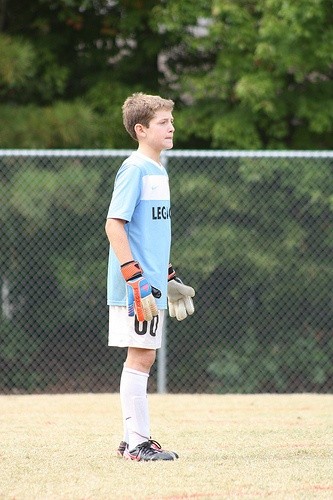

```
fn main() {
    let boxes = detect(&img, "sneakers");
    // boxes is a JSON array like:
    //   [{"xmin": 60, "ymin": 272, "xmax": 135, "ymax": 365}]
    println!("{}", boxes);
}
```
[{"xmin": 116, "ymin": 441, "xmax": 179, "ymax": 461}]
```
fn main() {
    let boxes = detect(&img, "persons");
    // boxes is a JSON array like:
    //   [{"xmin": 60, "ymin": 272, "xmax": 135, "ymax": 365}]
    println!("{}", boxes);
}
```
[{"xmin": 102, "ymin": 92, "xmax": 195, "ymax": 464}]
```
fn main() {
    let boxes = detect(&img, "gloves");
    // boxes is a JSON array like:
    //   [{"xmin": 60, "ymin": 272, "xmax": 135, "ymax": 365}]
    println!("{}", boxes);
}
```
[
  {"xmin": 120, "ymin": 261, "xmax": 162, "ymax": 322},
  {"xmin": 167, "ymin": 263, "xmax": 196, "ymax": 321}
]
